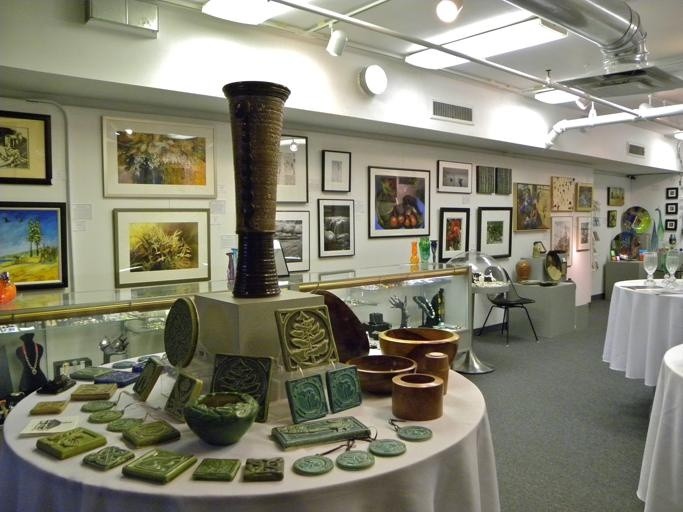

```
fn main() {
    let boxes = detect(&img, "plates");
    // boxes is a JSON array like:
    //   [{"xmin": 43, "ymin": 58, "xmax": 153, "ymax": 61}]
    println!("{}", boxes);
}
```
[
  {"xmin": 623, "ymin": 206, "xmax": 650, "ymax": 234},
  {"xmin": 544, "ymin": 249, "xmax": 562, "ymax": 282},
  {"xmin": 162, "ymin": 294, "xmax": 201, "ymax": 368},
  {"xmin": 309, "ymin": 287, "xmax": 370, "ymax": 363},
  {"xmin": 611, "ymin": 231, "xmax": 640, "ymax": 260}
]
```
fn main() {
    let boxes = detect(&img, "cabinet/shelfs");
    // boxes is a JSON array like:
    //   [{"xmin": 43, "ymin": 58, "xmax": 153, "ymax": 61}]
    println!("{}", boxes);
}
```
[{"xmin": 0, "ymin": 262, "xmax": 475, "ymax": 415}]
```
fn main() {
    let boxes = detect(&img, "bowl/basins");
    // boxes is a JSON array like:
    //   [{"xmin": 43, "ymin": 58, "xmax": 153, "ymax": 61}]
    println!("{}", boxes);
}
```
[{"xmin": 348, "ymin": 324, "xmax": 460, "ymax": 397}]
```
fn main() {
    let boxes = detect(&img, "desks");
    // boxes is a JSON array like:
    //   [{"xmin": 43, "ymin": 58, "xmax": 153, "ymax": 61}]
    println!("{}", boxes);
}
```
[{"xmin": 0, "ymin": 349, "xmax": 502, "ymax": 509}]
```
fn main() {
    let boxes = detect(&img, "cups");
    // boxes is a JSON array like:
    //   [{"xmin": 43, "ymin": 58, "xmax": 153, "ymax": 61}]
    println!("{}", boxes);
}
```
[
  {"xmin": 390, "ymin": 371, "xmax": 444, "ymax": 421},
  {"xmin": 184, "ymin": 391, "xmax": 258, "ymax": 447}
]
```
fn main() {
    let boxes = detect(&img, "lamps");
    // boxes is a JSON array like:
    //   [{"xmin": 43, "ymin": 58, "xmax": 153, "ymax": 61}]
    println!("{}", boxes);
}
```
[
  {"xmin": 434, "ymin": 0, "xmax": 462, "ymax": 24},
  {"xmin": 358, "ymin": 63, "xmax": 388, "ymax": 97},
  {"xmin": 403, "ymin": 16, "xmax": 570, "ymax": 71},
  {"xmin": 323, "ymin": 23, "xmax": 348, "ymax": 58}
]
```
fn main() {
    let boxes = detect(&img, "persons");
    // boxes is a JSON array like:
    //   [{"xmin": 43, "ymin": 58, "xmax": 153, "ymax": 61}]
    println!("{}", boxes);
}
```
[
  {"xmin": 388, "ymin": 294, "xmax": 410, "ymax": 329},
  {"xmin": 15, "ymin": 332, "xmax": 46, "ymax": 396}
]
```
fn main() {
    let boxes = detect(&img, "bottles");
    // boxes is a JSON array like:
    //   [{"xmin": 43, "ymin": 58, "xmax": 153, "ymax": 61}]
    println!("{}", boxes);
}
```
[
  {"xmin": 409, "ymin": 240, "xmax": 419, "ymax": 264},
  {"xmin": 532, "ymin": 241, "xmax": 541, "ymax": 258},
  {"xmin": 419, "ymin": 236, "xmax": 431, "ymax": 264}
]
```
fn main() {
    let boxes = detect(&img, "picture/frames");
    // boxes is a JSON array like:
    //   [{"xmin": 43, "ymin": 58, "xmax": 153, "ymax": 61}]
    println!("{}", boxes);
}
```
[
  {"xmin": 367, "ymin": 165, "xmax": 431, "ymax": 238},
  {"xmin": 436, "ymin": 160, "xmax": 591, "ymax": 267},
  {"xmin": 666, "ymin": 187, "xmax": 677, "ymax": 199},
  {"xmin": 100, "ymin": 115, "xmax": 217, "ymax": 201},
  {"xmin": 665, "ymin": 202, "xmax": 677, "ymax": 215},
  {"xmin": 665, "ymin": 219, "xmax": 677, "ymax": 230},
  {"xmin": 110, "ymin": 207, "xmax": 212, "ymax": 289},
  {"xmin": 0, "ymin": 110, "xmax": 52, "ymax": 186},
  {"xmin": 0, "ymin": 200, "xmax": 68, "ymax": 290},
  {"xmin": 275, "ymin": 134, "xmax": 356, "ymax": 271}
]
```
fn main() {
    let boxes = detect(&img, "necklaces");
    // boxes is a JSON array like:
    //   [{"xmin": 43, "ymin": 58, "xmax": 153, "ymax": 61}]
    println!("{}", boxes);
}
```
[
  {"xmin": 414, "ymin": 296, "xmax": 435, "ymax": 319},
  {"xmin": 21, "ymin": 342, "xmax": 40, "ymax": 376}
]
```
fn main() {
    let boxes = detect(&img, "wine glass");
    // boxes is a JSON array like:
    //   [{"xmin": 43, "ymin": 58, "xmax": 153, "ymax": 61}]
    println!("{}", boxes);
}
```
[
  {"xmin": 665, "ymin": 248, "xmax": 679, "ymax": 288},
  {"xmin": 431, "ymin": 239, "xmax": 438, "ymax": 264},
  {"xmin": 642, "ymin": 251, "xmax": 658, "ymax": 287}
]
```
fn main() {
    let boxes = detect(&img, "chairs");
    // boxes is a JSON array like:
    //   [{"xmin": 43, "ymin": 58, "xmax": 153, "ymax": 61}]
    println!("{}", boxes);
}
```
[{"xmin": 477, "ymin": 265, "xmax": 538, "ymax": 346}]
